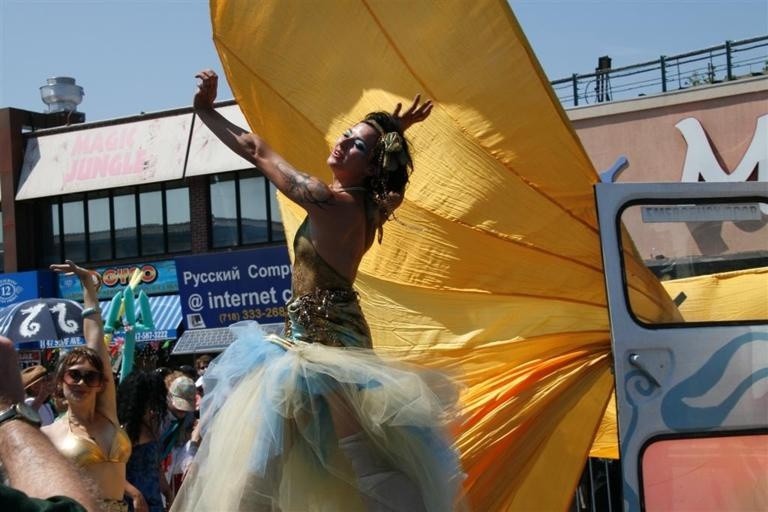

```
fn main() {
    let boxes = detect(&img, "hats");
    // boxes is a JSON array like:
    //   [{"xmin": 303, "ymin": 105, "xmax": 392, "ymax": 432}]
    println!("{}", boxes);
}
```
[
  {"xmin": 170, "ymin": 377, "xmax": 197, "ymax": 412},
  {"xmin": 20, "ymin": 363, "xmax": 49, "ymax": 389}
]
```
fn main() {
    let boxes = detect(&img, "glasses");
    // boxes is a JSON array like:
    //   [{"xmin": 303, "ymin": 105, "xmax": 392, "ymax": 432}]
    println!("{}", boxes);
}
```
[{"xmin": 62, "ymin": 368, "xmax": 105, "ymax": 387}]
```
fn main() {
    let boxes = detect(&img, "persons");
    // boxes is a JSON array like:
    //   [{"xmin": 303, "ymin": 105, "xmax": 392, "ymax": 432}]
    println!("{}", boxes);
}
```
[
  {"xmin": 1, "ymin": 260, "xmax": 213, "ymax": 511},
  {"xmin": 167, "ymin": 70, "xmax": 476, "ymax": 510}
]
